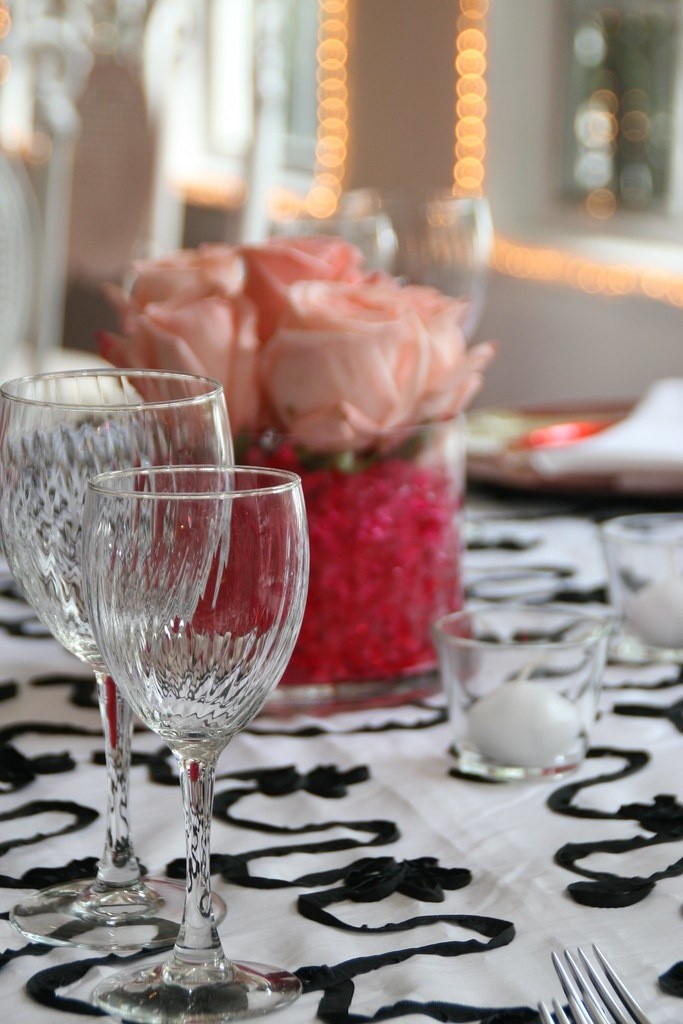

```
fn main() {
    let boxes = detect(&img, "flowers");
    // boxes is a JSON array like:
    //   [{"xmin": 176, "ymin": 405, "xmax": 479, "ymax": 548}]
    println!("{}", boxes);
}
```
[{"xmin": 97, "ymin": 231, "xmax": 498, "ymax": 469}]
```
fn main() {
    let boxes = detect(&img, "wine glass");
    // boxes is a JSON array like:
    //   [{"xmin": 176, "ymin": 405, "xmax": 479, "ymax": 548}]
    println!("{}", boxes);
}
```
[
  {"xmin": 0, "ymin": 369, "xmax": 236, "ymax": 953},
  {"xmin": 85, "ymin": 465, "xmax": 310, "ymax": 1024}
]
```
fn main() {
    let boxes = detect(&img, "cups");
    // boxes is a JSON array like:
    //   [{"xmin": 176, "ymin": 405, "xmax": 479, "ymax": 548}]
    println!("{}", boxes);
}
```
[
  {"xmin": 599, "ymin": 512, "xmax": 683, "ymax": 662},
  {"xmin": 430, "ymin": 604, "xmax": 613, "ymax": 783}
]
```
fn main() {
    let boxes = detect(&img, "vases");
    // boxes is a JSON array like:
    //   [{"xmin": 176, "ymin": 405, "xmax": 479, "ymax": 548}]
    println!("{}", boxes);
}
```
[{"xmin": 127, "ymin": 413, "xmax": 468, "ymax": 719}]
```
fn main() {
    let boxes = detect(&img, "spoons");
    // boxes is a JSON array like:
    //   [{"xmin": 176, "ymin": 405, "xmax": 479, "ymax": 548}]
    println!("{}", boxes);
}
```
[{"xmin": 537, "ymin": 943, "xmax": 652, "ymax": 1024}]
